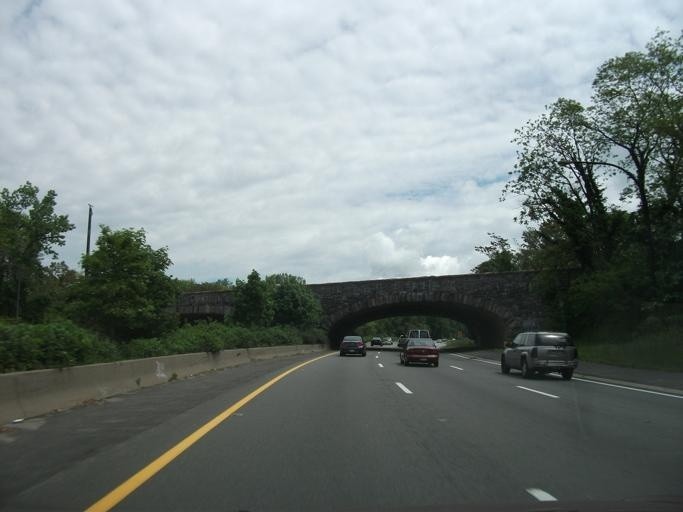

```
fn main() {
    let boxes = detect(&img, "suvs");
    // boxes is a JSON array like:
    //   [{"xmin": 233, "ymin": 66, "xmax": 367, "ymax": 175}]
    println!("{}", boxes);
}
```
[{"xmin": 499, "ymin": 329, "xmax": 580, "ymax": 382}]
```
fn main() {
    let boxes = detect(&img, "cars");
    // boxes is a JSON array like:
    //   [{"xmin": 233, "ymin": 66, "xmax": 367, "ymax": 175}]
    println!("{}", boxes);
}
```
[
  {"xmin": 369, "ymin": 328, "xmax": 447, "ymax": 368},
  {"xmin": 339, "ymin": 335, "xmax": 366, "ymax": 357}
]
